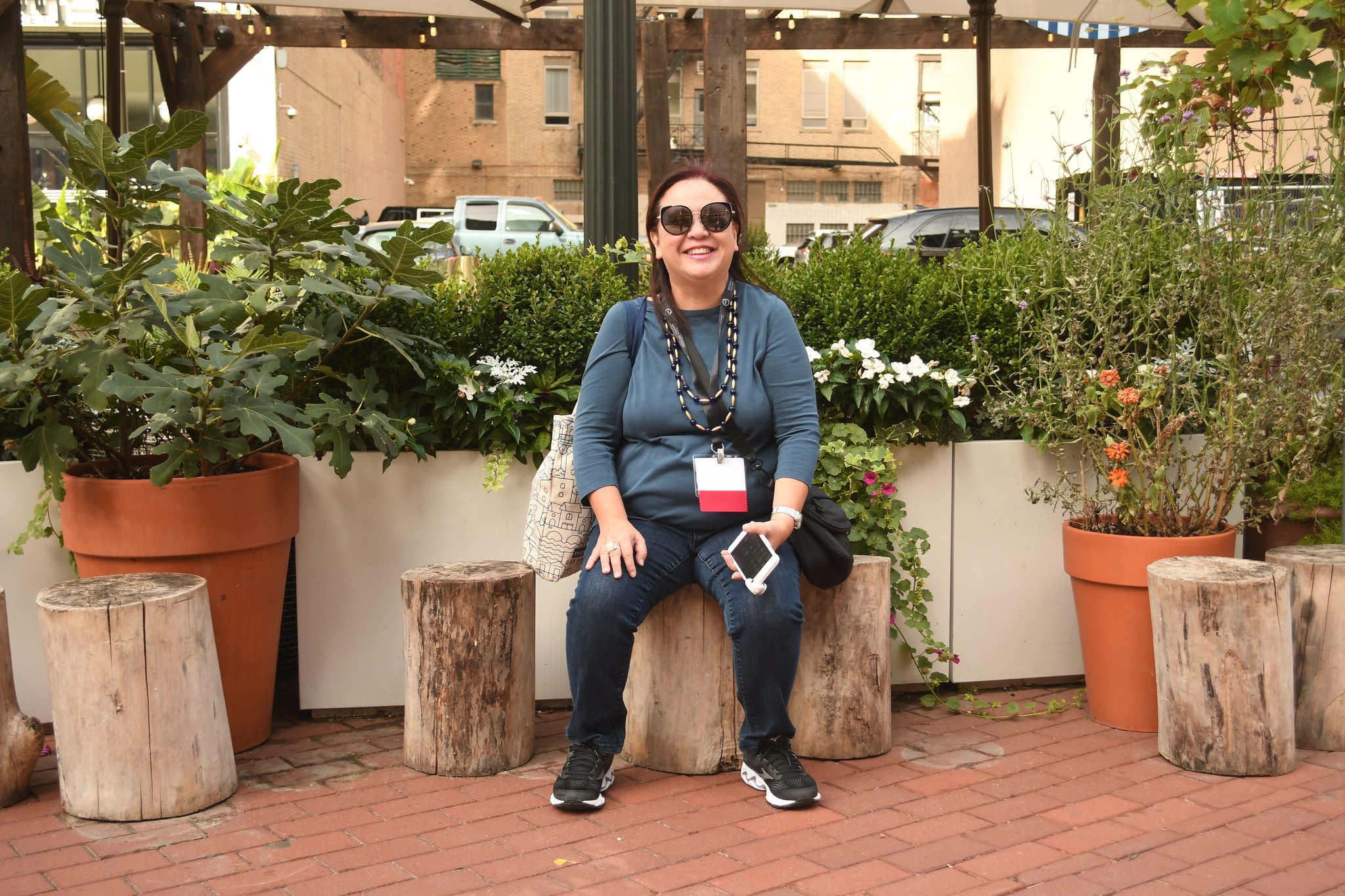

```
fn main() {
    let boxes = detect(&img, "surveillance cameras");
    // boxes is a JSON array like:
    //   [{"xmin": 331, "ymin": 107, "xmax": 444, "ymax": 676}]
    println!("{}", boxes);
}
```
[
  {"xmin": 408, "ymin": 180, "xmax": 416, "ymax": 185},
  {"xmin": 286, "ymin": 107, "xmax": 296, "ymax": 118}
]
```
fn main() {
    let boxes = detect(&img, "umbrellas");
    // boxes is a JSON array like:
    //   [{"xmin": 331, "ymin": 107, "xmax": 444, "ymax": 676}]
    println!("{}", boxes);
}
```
[
  {"xmin": 106, "ymin": 0, "xmax": 530, "ymax": 265},
  {"xmin": 520, "ymin": 0, "xmax": 1308, "ymax": 248}
]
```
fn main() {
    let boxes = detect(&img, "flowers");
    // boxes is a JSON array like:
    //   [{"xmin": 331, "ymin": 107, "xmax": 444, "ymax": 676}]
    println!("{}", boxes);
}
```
[{"xmin": 944, "ymin": 52, "xmax": 1345, "ymax": 549}]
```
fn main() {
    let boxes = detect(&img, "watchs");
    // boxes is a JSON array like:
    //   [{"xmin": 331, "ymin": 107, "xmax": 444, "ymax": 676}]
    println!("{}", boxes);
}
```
[{"xmin": 771, "ymin": 506, "xmax": 803, "ymax": 529}]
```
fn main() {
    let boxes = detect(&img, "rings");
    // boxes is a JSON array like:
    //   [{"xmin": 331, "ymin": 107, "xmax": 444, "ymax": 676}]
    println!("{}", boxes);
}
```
[{"xmin": 606, "ymin": 541, "xmax": 621, "ymax": 553}]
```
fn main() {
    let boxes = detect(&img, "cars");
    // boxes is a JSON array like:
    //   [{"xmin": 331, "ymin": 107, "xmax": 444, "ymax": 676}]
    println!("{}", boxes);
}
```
[{"xmin": 353, "ymin": 218, "xmax": 455, "ymax": 267}]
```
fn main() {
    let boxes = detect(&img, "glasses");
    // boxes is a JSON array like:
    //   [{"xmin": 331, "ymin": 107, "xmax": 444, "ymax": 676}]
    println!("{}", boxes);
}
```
[{"xmin": 655, "ymin": 201, "xmax": 736, "ymax": 236}]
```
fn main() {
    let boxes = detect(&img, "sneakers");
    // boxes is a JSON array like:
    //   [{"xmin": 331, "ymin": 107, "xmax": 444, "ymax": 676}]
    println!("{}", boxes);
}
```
[
  {"xmin": 550, "ymin": 743, "xmax": 615, "ymax": 810},
  {"xmin": 741, "ymin": 741, "xmax": 821, "ymax": 809}
]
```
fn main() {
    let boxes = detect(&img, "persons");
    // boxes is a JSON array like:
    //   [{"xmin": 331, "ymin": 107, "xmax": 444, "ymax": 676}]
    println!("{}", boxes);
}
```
[{"xmin": 549, "ymin": 163, "xmax": 821, "ymax": 809}]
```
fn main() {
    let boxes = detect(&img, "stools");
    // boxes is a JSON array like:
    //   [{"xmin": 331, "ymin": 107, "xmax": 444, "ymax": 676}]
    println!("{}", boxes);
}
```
[
  {"xmin": 400, "ymin": 557, "xmax": 539, "ymax": 781},
  {"xmin": 1148, "ymin": 555, "xmax": 1296, "ymax": 775},
  {"xmin": 1263, "ymin": 542, "xmax": 1345, "ymax": 754},
  {"xmin": 618, "ymin": 577, "xmax": 745, "ymax": 776},
  {"xmin": 35, "ymin": 562, "xmax": 246, "ymax": 820},
  {"xmin": 784, "ymin": 549, "xmax": 894, "ymax": 762}
]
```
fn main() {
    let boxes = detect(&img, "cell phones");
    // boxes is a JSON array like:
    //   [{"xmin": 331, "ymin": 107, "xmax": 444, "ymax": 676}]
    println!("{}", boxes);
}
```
[{"xmin": 728, "ymin": 522, "xmax": 780, "ymax": 587}]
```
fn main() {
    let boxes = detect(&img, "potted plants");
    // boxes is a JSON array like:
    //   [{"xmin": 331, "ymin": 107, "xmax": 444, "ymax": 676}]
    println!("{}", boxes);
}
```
[{"xmin": 0, "ymin": 55, "xmax": 498, "ymax": 753}]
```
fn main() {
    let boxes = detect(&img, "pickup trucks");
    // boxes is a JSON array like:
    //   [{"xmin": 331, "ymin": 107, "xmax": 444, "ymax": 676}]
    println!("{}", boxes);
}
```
[{"xmin": 453, "ymin": 196, "xmax": 585, "ymax": 258}]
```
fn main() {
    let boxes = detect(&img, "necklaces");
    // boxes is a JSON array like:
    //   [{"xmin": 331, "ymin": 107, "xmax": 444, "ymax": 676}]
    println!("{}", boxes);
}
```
[{"xmin": 663, "ymin": 288, "xmax": 740, "ymax": 433}]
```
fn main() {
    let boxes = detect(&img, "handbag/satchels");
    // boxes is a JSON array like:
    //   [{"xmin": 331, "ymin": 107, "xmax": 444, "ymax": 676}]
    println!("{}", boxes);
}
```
[
  {"xmin": 762, "ymin": 475, "xmax": 855, "ymax": 590},
  {"xmin": 523, "ymin": 391, "xmax": 596, "ymax": 582}
]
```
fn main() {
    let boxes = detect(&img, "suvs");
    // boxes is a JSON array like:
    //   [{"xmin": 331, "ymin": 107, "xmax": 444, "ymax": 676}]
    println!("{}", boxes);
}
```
[{"xmin": 791, "ymin": 204, "xmax": 1091, "ymax": 266}]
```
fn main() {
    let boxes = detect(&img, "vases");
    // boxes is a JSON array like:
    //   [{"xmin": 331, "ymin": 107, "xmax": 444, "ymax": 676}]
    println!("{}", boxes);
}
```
[
  {"xmin": 1060, "ymin": 511, "xmax": 1238, "ymax": 736},
  {"xmin": 1237, "ymin": 487, "xmax": 1345, "ymax": 561}
]
